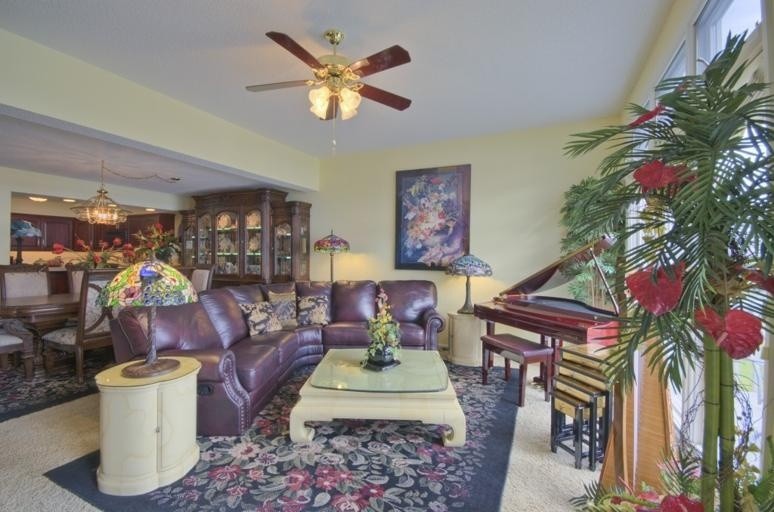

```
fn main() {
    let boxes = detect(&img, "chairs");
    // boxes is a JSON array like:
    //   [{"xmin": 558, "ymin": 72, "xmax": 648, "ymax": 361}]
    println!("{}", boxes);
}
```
[
  {"xmin": 31, "ymin": 264, "xmax": 121, "ymax": 385},
  {"xmin": 0, "ymin": 317, "xmax": 38, "ymax": 386},
  {"xmin": 0, "ymin": 261, "xmax": 67, "ymax": 338},
  {"xmin": 61, "ymin": 262, "xmax": 87, "ymax": 299},
  {"xmin": 184, "ymin": 260, "xmax": 217, "ymax": 295}
]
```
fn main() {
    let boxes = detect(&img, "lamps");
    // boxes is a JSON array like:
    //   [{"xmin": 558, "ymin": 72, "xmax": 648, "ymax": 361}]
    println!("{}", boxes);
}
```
[
  {"xmin": 443, "ymin": 243, "xmax": 495, "ymax": 315},
  {"xmin": 91, "ymin": 259, "xmax": 201, "ymax": 378},
  {"xmin": 306, "ymin": 73, "xmax": 360, "ymax": 119},
  {"xmin": 68, "ymin": 157, "xmax": 133, "ymax": 227},
  {"xmin": 9, "ymin": 219, "xmax": 43, "ymax": 264},
  {"xmin": 315, "ymin": 230, "xmax": 350, "ymax": 284}
]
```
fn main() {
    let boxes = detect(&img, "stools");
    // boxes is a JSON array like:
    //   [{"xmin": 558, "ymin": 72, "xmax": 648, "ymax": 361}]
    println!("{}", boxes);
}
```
[{"xmin": 479, "ymin": 330, "xmax": 552, "ymax": 407}]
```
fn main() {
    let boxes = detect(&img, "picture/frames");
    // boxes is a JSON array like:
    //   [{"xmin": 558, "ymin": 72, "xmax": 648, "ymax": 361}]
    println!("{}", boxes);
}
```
[{"xmin": 391, "ymin": 163, "xmax": 472, "ymax": 272}]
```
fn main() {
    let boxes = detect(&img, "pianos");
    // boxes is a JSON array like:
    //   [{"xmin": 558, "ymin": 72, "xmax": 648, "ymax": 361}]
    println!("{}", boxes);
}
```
[{"xmin": 474, "ymin": 236, "xmax": 623, "ymax": 386}]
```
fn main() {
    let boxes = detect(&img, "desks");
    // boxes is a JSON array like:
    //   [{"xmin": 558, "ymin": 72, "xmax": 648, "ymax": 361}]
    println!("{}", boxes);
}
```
[
  {"xmin": 1, "ymin": 291, "xmax": 85, "ymax": 381},
  {"xmin": 550, "ymin": 343, "xmax": 625, "ymax": 469}
]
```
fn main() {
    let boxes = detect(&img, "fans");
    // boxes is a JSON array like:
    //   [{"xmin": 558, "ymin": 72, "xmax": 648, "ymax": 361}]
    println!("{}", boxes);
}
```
[{"xmin": 244, "ymin": 28, "xmax": 416, "ymax": 119}]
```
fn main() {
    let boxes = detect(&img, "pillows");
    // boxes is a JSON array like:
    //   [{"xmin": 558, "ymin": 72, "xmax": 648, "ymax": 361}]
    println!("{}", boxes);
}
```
[
  {"xmin": 266, "ymin": 290, "xmax": 297, "ymax": 329},
  {"xmin": 296, "ymin": 292, "xmax": 328, "ymax": 327},
  {"xmin": 236, "ymin": 300, "xmax": 281, "ymax": 338}
]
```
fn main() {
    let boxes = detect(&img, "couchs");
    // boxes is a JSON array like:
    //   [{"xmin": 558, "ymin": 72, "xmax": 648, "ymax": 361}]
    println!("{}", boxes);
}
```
[{"xmin": 108, "ymin": 278, "xmax": 444, "ymax": 438}]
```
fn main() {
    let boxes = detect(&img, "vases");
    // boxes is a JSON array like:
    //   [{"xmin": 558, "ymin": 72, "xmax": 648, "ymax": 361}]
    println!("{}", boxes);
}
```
[{"xmin": 364, "ymin": 346, "xmax": 396, "ymax": 364}]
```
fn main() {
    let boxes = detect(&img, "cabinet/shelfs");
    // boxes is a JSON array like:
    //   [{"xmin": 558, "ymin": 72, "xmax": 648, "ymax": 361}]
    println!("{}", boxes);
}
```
[
  {"xmin": 444, "ymin": 312, "xmax": 488, "ymax": 369},
  {"xmin": 190, "ymin": 187, "xmax": 288, "ymax": 289},
  {"xmin": 73, "ymin": 212, "xmax": 98, "ymax": 255},
  {"xmin": 124, "ymin": 211, "xmax": 175, "ymax": 251},
  {"xmin": 271, "ymin": 199, "xmax": 311, "ymax": 284},
  {"xmin": 92, "ymin": 353, "xmax": 202, "ymax": 497},
  {"xmin": 93, "ymin": 210, "xmax": 126, "ymax": 251},
  {"xmin": 178, "ymin": 208, "xmax": 195, "ymax": 275},
  {"xmin": 10, "ymin": 210, "xmax": 72, "ymax": 251}
]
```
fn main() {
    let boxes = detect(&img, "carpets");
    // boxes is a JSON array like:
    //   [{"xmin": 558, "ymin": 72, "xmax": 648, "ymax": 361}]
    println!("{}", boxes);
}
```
[
  {"xmin": 40, "ymin": 357, "xmax": 532, "ymax": 512},
  {"xmin": 1, "ymin": 337, "xmax": 114, "ymax": 421}
]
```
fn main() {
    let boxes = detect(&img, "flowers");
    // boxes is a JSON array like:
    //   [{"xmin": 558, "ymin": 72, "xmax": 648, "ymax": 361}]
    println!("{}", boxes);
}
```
[
  {"xmin": 362, "ymin": 288, "xmax": 402, "ymax": 350},
  {"xmin": 50, "ymin": 237, "xmax": 134, "ymax": 271},
  {"xmin": 613, "ymin": 102, "xmax": 771, "ymax": 510},
  {"xmin": 134, "ymin": 221, "xmax": 183, "ymax": 260}
]
